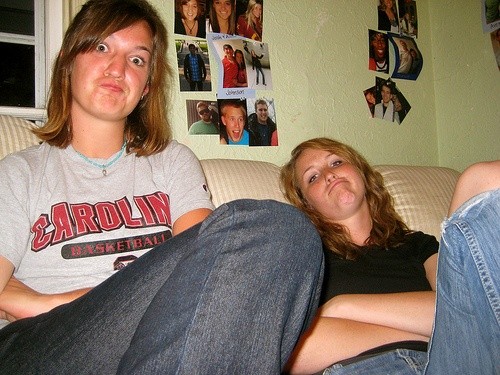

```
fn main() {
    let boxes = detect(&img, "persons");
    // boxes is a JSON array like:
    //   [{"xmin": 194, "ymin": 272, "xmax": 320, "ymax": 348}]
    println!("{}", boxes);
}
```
[
  {"xmin": 369, "ymin": 33, "xmax": 389, "ymax": 74},
  {"xmin": 175, "ymin": 0, "xmax": 206, "ymax": 38},
  {"xmin": 365, "ymin": 83, "xmax": 407, "ymax": 126},
  {"xmin": 189, "ymin": 102, "xmax": 220, "ymax": 134},
  {"xmin": 251, "ymin": 50, "xmax": 267, "ymax": 86},
  {"xmin": 206, "ymin": 0, "xmax": 236, "ymax": 36},
  {"xmin": 279, "ymin": 137, "xmax": 500, "ymax": 375},
  {"xmin": 0, "ymin": 0, "xmax": 324, "ymax": 375},
  {"xmin": 396, "ymin": 40, "xmax": 421, "ymax": 76},
  {"xmin": 222, "ymin": 44, "xmax": 248, "ymax": 88},
  {"xmin": 377, "ymin": 0, "xmax": 415, "ymax": 36},
  {"xmin": 219, "ymin": 102, "xmax": 249, "ymax": 145},
  {"xmin": 184, "ymin": 44, "xmax": 207, "ymax": 91},
  {"xmin": 247, "ymin": 99, "xmax": 278, "ymax": 146},
  {"xmin": 236, "ymin": 0, "xmax": 262, "ymax": 42}
]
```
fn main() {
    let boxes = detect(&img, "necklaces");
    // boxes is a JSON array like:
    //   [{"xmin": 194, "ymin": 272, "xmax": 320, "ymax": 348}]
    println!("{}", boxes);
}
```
[
  {"xmin": 184, "ymin": 20, "xmax": 196, "ymax": 34},
  {"xmin": 75, "ymin": 138, "xmax": 127, "ymax": 175}
]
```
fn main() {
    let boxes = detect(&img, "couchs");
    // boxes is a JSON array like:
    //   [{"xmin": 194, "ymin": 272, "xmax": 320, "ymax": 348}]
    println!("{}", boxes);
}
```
[{"xmin": 0, "ymin": 114, "xmax": 461, "ymax": 242}]
